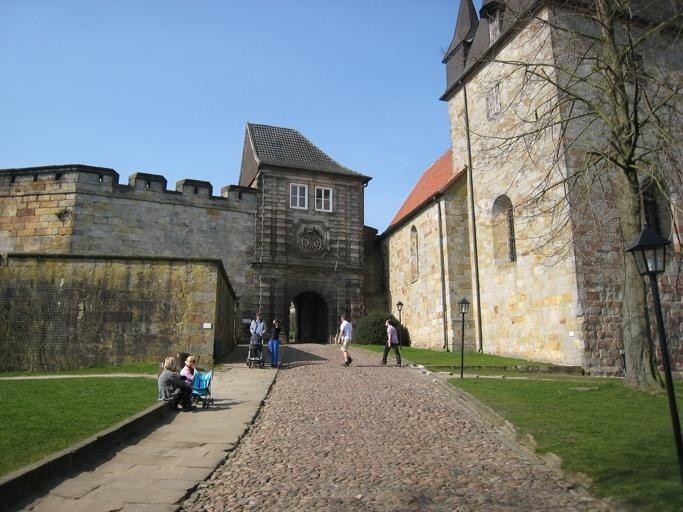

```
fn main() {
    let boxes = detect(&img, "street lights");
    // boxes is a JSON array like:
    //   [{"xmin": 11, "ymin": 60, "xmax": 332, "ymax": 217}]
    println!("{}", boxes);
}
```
[
  {"xmin": 395, "ymin": 300, "xmax": 404, "ymax": 350},
  {"xmin": 456, "ymin": 297, "xmax": 471, "ymax": 379},
  {"xmin": 624, "ymin": 225, "xmax": 682, "ymax": 483}
]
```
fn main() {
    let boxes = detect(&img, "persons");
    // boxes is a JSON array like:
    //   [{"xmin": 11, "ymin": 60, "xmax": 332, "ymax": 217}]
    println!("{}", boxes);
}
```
[
  {"xmin": 249, "ymin": 312, "xmax": 266, "ymax": 359},
  {"xmin": 378, "ymin": 319, "xmax": 402, "ymax": 367},
  {"xmin": 336, "ymin": 313, "xmax": 353, "ymax": 367},
  {"xmin": 157, "ymin": 356, "xmax": 196, "ymax": 412},
  {"xmin": 178, "ymin": 356, "xmax": 199, "ymax": 402},
  {"xmin": 267, "ymin": 318, "xmax": 282, "ymax": 368}
]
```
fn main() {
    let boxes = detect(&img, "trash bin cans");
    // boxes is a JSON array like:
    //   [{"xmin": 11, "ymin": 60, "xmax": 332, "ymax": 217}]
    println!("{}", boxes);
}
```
[{"xmin": 175, "ymin": 353, "xmax": 189, "ymax": 369}]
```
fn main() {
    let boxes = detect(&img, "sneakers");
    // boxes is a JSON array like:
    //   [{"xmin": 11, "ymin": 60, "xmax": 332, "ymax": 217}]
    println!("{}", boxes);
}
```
[
  {"xmin": 395, "ymin": 364, "xmax": 401, "ymax": 367},
  {"xmin": 380, "ymin": 361, "xmax": 387, "ymax": 364}
]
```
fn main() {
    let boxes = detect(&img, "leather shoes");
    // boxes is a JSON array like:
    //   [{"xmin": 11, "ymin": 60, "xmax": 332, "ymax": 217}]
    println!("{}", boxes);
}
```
[
  {"xmin": 177, "ymin": 404, "xmax": 196, "ymax": 412},
  {"xmin": 341, "ymin": 357, "xmax": 352, "ymax": 366}
]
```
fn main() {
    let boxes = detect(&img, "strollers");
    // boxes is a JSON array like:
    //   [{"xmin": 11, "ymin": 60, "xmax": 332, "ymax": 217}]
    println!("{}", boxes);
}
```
[
  {"xmin": 190, "ymin": 369, "xmax": 215, "ymax": 409},
  {"xmin": 247, "ymin": 332, "xmax": 265, "ymax": 368}
]
